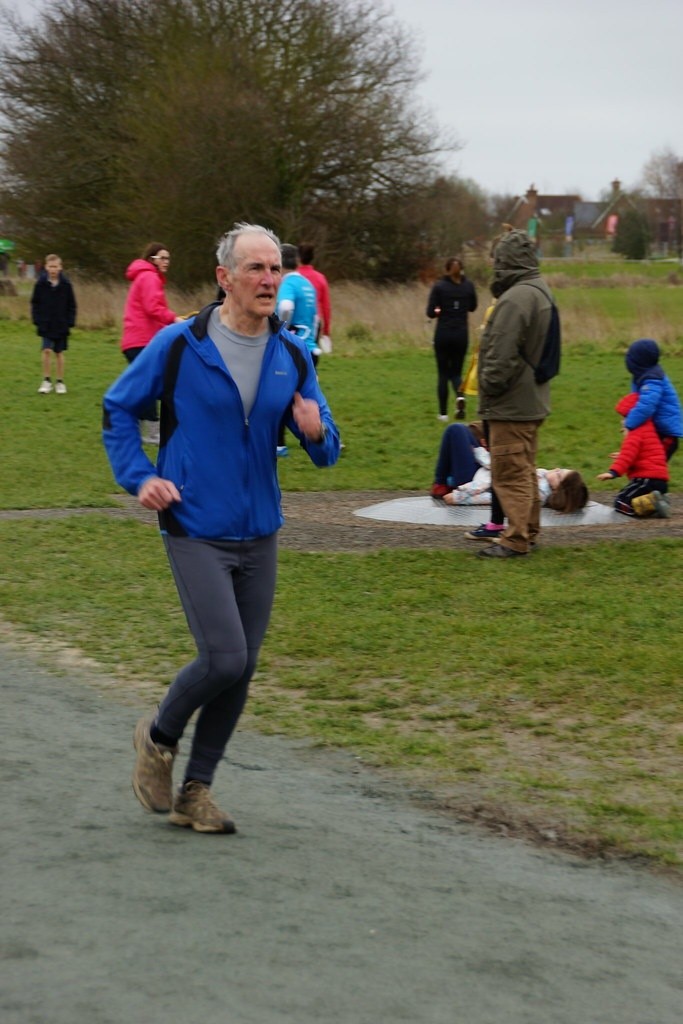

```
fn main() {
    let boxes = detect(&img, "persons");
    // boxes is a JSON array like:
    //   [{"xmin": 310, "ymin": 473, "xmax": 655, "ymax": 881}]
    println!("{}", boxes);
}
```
[
  {"xmin": 121, "ymin": 242, "xmax": 200, "ymax": 446},
  {"xmin": 102, "ymin": 221, "xmax": 341, "ymax": 832},
  {"xmin": 426, "ymin": 258, "xmax": 478, "ymax": 421},
  {"xmin": 477, "ymin": 231, "xmax": 557, "ymax": 560},
  {"xmin": 596, "ymin": 392, "xmax": 671, "ymax": 520},
  {"xmin": 464, "ymin": 238, "xmax": 506, "ymax": 542},
  {"xmin": 297, "ymin": 241, "xmax": 332, "ymax": 367},
  {"xmin": 430, "ymin": 425, "xmax": 589, "ymax": 516},
  {"xmin": 274, "ymin": 245, "xmax": 346, "ymax": 457},
  {"xmin": 621, "ymin": 338, "xmax": 683, "ymax": 462},
  {"xmin": 30, "ymin": 254, "xmax": 77, "ymax": 394}
]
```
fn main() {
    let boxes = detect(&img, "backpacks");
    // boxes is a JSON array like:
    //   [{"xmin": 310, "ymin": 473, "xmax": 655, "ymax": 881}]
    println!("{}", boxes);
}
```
[{"xmin": 515, "ymin": 280, "xmax": 562, "ymax": 386}]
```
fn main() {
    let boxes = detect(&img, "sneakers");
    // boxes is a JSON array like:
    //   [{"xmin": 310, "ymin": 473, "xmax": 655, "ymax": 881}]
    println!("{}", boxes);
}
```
[
  {"xmin": 132, "ymin": 716, "xmax": 176, "ymax": 816},
  {"xmin": 170, "ymin": 781, "xmax": 234, "ymax": 834},
  {"xmin": 463, "ymin": 524, "xmax": 505, "ymax": 542},
  {"xmin": 477, "ymin": 539, "xmax": 538, "ymax": 558}
]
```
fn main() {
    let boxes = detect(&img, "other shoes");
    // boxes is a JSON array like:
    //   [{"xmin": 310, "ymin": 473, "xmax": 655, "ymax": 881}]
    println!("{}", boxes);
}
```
[
  {"xmin": 436, "ymin": 414, "xmax": 448, "ymax": 422},
  {"xmin": 56, "ymin": 382, "xmax": 66, "ymax": 393},
  {"xmin": 340, "ymin": 439, "xmax": 345, "ymax": 450},
  {"xmin": 38, "ymin": 379, "xmax": 53, "ymax": 392},
  {"xmin": 651, "ymin": 490, "xmax": 673, "ymax": 518},
  {"xmin": 139, "ymin": 418, "xmax": 160, "ymax": 444},
  {"xmin": 454, "ymin": 397, "xmax": 466, "ymax": 421},
  {"xmin": 432, "ymin": 485, "xmax": 451, "ymax": 499},
  {"xmin": 277, "ymin": 447, "xmax": 289, "ymax": 457}
]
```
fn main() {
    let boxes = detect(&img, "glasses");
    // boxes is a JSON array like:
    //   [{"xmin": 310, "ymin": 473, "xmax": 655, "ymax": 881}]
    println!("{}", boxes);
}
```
[{"xmin": 150, "ymin": 255, "xmax": 171, "ymax": 261}]
